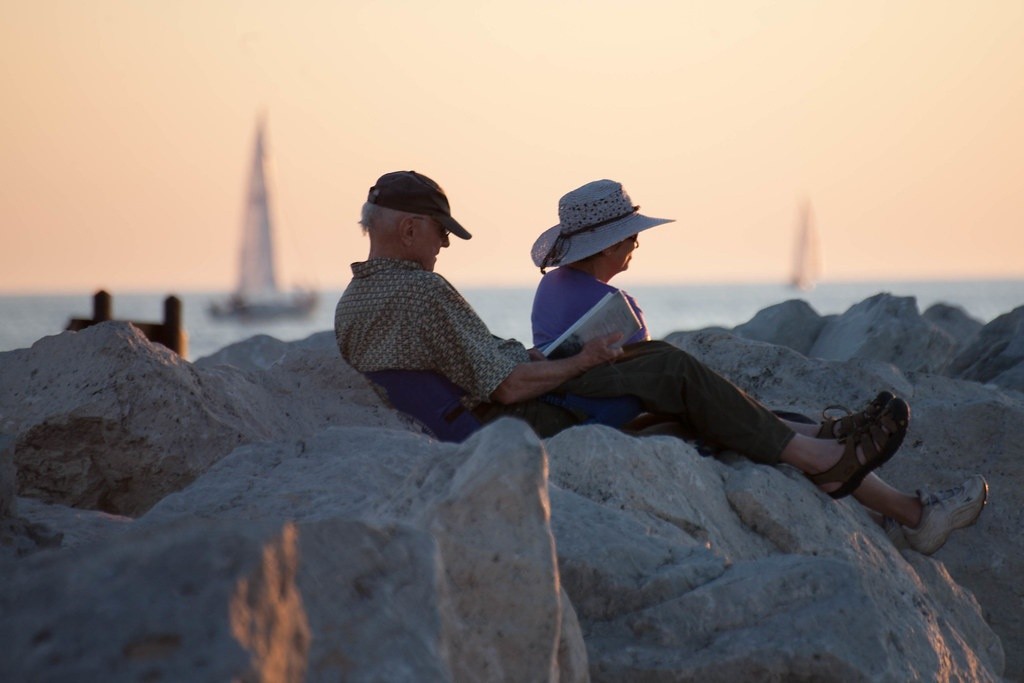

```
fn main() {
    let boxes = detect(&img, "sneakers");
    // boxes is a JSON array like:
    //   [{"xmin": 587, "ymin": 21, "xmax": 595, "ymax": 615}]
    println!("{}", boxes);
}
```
[
  {"xmin": 885, "ymin": 518, "xmax": 908, "ymax": 549},
  {"xmin": 900, "ymin": 475, "xmax": 988, "ymax": 555}
]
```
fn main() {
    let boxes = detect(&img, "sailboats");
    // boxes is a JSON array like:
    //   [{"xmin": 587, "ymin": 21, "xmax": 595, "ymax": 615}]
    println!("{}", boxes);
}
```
[{"xmin": 200, "ymin": 112, "xmax": 323, "ymax": 327}]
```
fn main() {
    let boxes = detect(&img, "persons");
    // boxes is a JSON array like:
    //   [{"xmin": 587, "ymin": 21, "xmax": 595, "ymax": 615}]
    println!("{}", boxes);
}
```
[
  {"xmin": 531, "ymin": 180, "xmax": 989, "ymax": 555},
  {"xmin": 333, "ymin": 170, "xmax": 912, "ymax": 501}
]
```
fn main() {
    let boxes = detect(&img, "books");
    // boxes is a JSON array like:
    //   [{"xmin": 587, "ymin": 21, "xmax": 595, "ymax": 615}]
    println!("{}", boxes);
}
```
[{"xmin": 538, "ymin": 290, "xmax": 644, "ymax": 361}]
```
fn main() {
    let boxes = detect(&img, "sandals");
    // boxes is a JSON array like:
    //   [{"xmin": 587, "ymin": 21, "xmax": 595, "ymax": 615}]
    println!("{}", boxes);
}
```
[
  {"xmin": 804, "ymin": 397, "xmax": 911, "ymax": 499},
  {"xmin": 816, "ymin": 390, "xmax": 895, "ymax": 440}
]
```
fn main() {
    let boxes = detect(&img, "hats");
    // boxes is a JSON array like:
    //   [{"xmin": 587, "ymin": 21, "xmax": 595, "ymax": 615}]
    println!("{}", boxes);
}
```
[
  {"xmin": 369, "ymin": 170, "xmax": 473, "ymax": 239},
  {"xmin": 531, "ymin": 179, "xmax": 675, "ymax": 274}
]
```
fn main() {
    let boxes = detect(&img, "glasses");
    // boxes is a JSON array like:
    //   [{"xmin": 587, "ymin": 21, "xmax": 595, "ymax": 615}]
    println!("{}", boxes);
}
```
[{"xmin": 413, "ymin": 216, "xmax": 450, "ymax": 237}]
